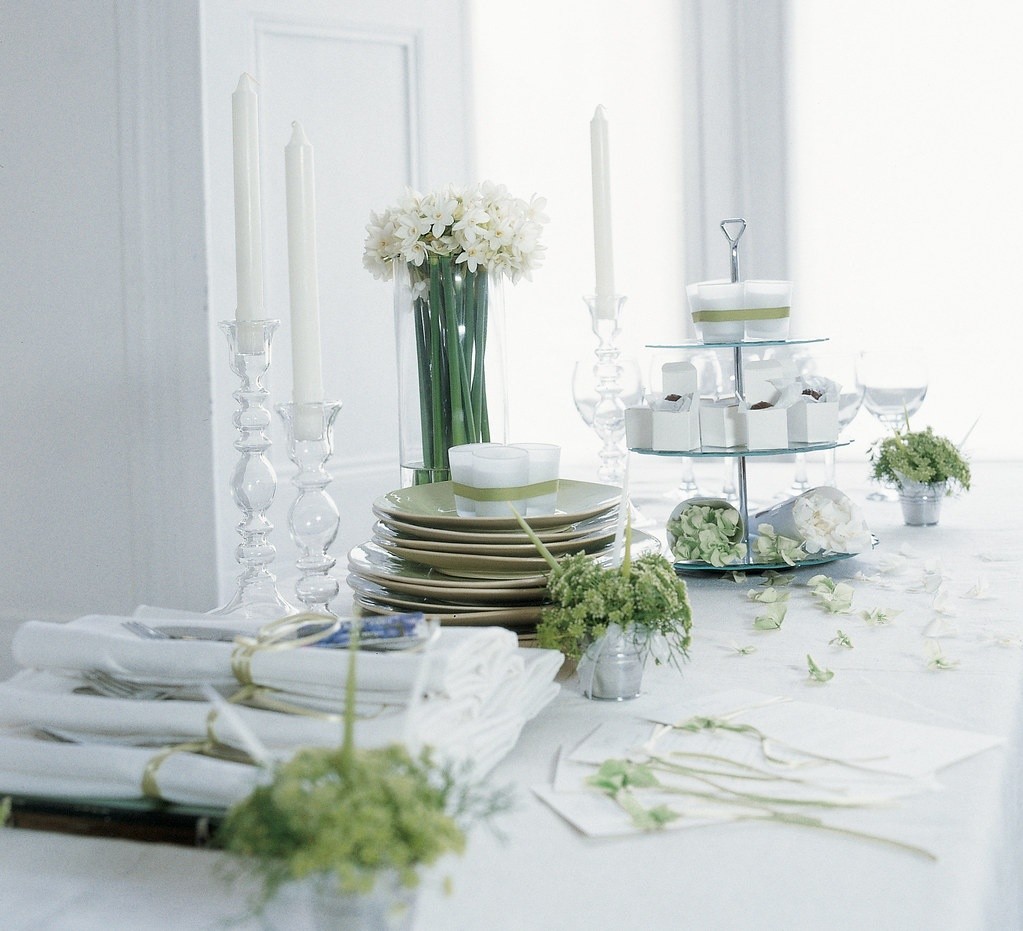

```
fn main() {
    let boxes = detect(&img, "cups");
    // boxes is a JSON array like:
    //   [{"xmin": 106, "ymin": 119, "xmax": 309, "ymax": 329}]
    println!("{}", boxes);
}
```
[
  {"xmin": 747, "ymin": 486, "xmax": 869, "ymax": 554},
  {"xmin": 685, "ymin": 278, "xmax": 793, "ymax": 346},
  {"xmin": 447, "ymin": 439, "xmax": 561, "ymax": 519},
  {"xmin": 665, "ymin": 496, "xmax": 745, "ymax": 561}
]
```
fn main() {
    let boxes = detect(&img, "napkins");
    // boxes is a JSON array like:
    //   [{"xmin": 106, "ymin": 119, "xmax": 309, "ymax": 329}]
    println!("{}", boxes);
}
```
[{"xmin": 0, "ymin": 603, "xmax": 566, "ymax": 809}]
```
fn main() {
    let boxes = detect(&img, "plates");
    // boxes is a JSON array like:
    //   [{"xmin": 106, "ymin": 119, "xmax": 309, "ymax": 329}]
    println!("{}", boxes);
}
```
[
  {"xmin": 346, "ymin": 480, "xmax": 663, "ymax": 640},
  {"xmin": 672, "ymin": 532, "xmax": 880, "ymax": 573}
]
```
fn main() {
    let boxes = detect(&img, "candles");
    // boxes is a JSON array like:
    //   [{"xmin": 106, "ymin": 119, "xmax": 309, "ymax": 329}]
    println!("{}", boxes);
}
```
[
  {"xmin": 232, "ymin": 74, "xmax": 263, "ymax": 353},
  {"xmin": 283, "ymin": 120, "xmax": 326, "ymax": 436},
  {"xmin": 589, "ymin": 105, "xmax": 616, "ymax": 318}
]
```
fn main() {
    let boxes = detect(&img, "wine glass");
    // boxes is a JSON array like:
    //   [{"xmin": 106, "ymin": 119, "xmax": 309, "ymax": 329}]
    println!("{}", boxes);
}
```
[
  {"xmin": 852, "ymin": 342, "xmax": 930, "ymax": 501},
  {"xmin": 571, "ymin": 346, "xmax": 645, "ymax": 521},
  {"xmin": 646, "ymin": 340, "xmax": 867, "ymax": 512}
]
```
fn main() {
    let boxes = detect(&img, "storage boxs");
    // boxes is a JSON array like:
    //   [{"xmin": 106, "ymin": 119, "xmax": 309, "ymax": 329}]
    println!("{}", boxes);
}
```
[{"xmin": 625, "ymin": 360, "xmax": 842, "ymax": 452}]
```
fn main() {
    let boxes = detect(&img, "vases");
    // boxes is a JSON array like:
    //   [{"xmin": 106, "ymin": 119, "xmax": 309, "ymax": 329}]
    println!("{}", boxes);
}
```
[
  {"xmin": 583, "ymin": 620, "xmax": 653, "ymax": 702},
  {"xmin": 891, "ymin": 469, "xmax": 947, "ymax": 526},
  {"xmin": 394, "ymin": 256, "xmax": 506, "ymax": 490},
  {"xmin": 303, "ymin": 869, "xmax": 420, "ymax": 931}
]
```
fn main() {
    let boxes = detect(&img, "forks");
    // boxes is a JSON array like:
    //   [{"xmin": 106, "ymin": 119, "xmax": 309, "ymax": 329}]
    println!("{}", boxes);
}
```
[
  {"xmin": 121, "ymin": 617, "xmax": 235, "ymax": 645},
  {"xmin": 82, "ymin": 666, "xmax": 339, "ymax": 720}
]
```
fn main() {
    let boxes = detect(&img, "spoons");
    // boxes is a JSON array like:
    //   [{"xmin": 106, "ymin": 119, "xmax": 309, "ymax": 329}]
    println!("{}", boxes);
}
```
[{"xmin": 36, "ymin": 723, "xmax": 233, "ymax": 749}]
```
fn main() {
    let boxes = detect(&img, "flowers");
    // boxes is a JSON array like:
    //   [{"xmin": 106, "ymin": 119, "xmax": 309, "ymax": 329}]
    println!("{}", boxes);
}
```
[
  {"xmin": 199, "ymin": 600, "xmax": 470, "ymax": 912},
  {"xmin": 507, "ymin": 490, "xmax": 693, "ymax": 677},
  {"xmin": 360, "ymin": 180, "xmax": 550, "ymax": 485},
  {"xmin": 865, "ymin": 397, "xmax": 979, "ymax": 495},
  {"xmin": 588, "ymin": 715, "xmax": 938, "ymax": 864},
  {"xmin": 669, "ymin": 494, "xmax": 1023, "ymax": 684}
]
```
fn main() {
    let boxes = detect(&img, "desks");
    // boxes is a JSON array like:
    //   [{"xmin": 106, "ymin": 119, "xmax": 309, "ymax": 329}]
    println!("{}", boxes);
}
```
[{"xmin": 0, "ymin": 476, "xmax": 1022, "ymax": 929}]
